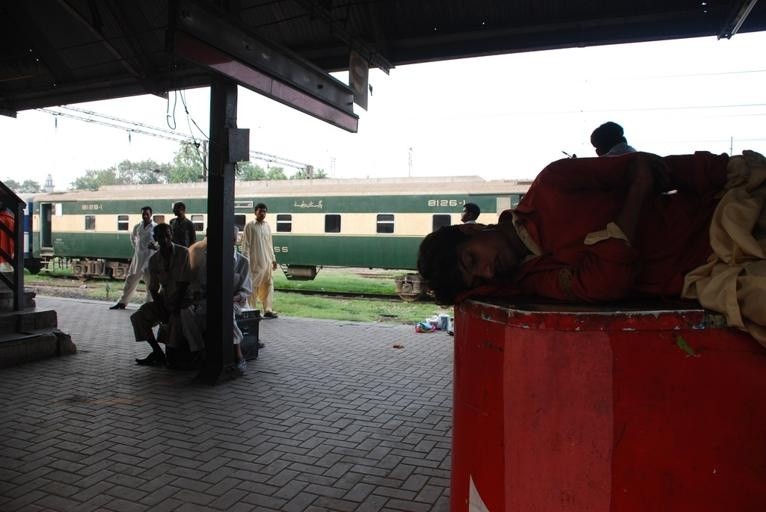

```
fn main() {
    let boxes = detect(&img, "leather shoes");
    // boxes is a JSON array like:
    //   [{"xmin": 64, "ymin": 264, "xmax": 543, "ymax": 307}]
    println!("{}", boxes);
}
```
[
  {"xmin": 110, "ymin": 303, "xmax": 125, "ymax": 309},
  {"xmin": 135, "ymin": 352, "xmax": 165, "ymax": 362}
]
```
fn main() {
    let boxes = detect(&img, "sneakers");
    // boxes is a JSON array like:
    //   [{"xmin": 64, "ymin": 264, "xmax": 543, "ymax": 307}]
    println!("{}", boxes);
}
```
[
  {"xmin": 238, "ymin": 359, "xmax": 247, "ymax": 369},
  {"xmin": 264, "ymin": 312, "xmax": 277, "ymax": 318}
]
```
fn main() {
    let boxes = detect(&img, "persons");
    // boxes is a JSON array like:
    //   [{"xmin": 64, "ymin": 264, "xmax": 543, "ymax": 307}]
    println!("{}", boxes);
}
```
[
  {"xmin": 168, "ymin": 202, "xmax": 197, "ymax": 249},
  {"xmin": 417, "ymin": 152, "xmax": 766, "ymax": 351},
  {"xmin": 182, "ymin": 226, "xmax": 249, "ymax": 382},
  {"xmin": 165, "ymin": 226, "xmax": 208, "ymax": 372},
  {"xmin": 242, "ymin": 204, "xmax": 279, "ymax": 320},
  {"xmin": 130, "ymin": 222, "xmax": 191, "ymax": 369},
  {"xmin": 109, "ymin": 207, "xmax": 161, "ymax": 310},
  {"xmin": 567, "ymin": 121, "xmax": 639, "ymax": 160},
  {"xmin": 0, "ymin": 197, "xmax": 16, "ymax": 273},
  {"xmin": 459, "ymin": 202, "xmax": 483, "ymax": 226}
]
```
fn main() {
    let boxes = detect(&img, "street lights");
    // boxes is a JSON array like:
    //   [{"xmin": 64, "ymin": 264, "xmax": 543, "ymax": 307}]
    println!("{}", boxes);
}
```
[{"xmin": 408, "ymin": 147, "xmax": 413, "ymax": 176}]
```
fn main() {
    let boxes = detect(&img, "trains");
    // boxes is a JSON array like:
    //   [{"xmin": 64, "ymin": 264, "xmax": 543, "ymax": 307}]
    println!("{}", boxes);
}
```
[{"xmin": 12, "ymin": 168, "xmax": 534, "ymax": 306}]
